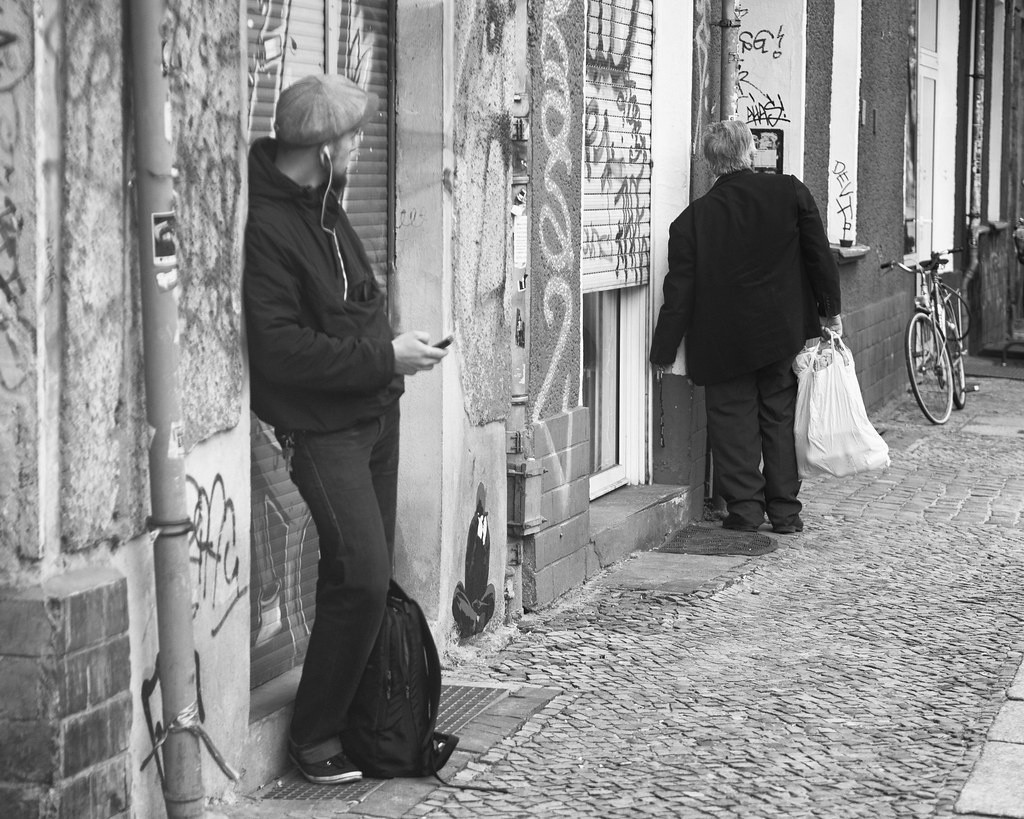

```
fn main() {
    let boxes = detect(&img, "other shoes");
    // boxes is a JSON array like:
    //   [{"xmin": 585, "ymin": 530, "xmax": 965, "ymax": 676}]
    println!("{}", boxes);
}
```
[
  {"xmin": 773, "ymin": 514, "xmax": 804, "ymax": 533},
  {"xmin": 723, "ymin": 514, "xmax": 759, "ymax": 531}
]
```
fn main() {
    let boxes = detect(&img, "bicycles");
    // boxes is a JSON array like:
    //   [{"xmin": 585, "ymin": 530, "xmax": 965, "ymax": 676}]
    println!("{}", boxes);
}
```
[{"xmin": 879, "ymin": 247, "xmax": 979, "ymax": 426}]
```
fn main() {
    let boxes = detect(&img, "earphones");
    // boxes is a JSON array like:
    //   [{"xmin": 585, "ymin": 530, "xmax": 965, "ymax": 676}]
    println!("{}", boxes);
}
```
[{"xmin": 323, "ymin": 145, "xmax": 330, "ymax": 159}]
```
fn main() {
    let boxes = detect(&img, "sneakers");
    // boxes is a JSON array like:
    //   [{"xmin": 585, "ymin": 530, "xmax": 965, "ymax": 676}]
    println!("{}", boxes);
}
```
[{"xmin": 288, "ymin": 734, "xmax": 361, "ymax": 785}]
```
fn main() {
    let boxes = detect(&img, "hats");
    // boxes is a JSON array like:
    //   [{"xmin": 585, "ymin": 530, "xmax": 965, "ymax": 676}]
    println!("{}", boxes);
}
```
[{"xmin": 274, "ymin": 74, "xmax": 379, "ymax": 145}]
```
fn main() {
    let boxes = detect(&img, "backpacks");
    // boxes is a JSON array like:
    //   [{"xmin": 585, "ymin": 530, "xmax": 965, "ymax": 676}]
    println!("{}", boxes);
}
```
[{"xmin": 339, "ymin": 578, "xmax": 459, "ymax": 779}]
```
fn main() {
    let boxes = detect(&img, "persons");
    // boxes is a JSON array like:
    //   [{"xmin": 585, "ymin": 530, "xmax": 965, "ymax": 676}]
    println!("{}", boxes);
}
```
[
  {"xmin": 243, "ymin": 74, "xmax": 447, "ymax": 783},
  {"xmin": 649, "ymin": 121, "xmax": 842, "ymax": 533}
]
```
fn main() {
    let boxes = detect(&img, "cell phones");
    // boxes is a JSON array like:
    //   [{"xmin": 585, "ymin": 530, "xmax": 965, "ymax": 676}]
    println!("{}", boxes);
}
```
[{"xmin": 433, "ymin": 335, "xmax": 453, "ymax": 349}]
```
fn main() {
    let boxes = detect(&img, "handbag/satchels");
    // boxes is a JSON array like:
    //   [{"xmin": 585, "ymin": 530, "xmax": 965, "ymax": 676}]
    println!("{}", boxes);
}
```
[{"xmin": 794, "ymin": 330, "xmax": 890, "ymax": 483}]
